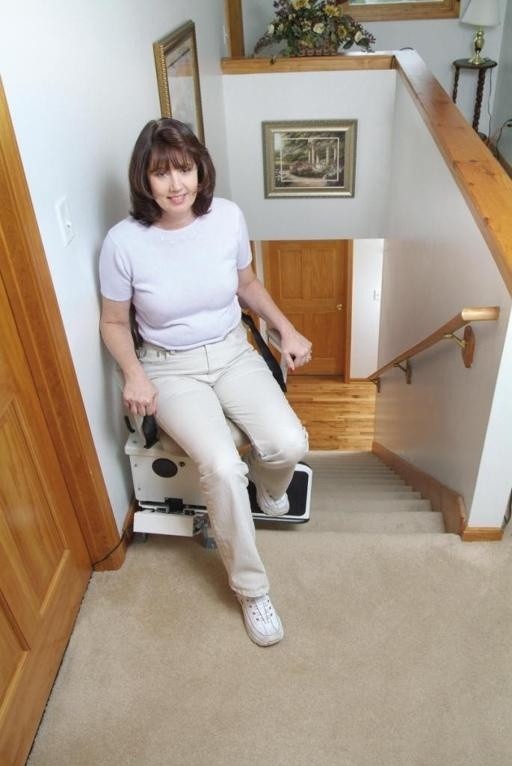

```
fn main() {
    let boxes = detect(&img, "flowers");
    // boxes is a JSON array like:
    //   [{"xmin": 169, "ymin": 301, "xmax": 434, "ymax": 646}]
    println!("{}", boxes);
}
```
[{"xmin": 247, "ymin": 0, "xmax": 376, "ymax": 66}]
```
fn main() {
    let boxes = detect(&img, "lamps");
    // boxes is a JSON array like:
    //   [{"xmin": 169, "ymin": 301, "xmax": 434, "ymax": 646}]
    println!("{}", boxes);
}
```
[{"xmin": 457, "ymin": 0, "xmax": 501, "ymax": 66}]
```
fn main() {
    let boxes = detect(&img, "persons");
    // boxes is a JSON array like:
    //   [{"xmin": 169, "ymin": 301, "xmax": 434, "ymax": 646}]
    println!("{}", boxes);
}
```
[{"xmin": 100, "ymin": 118, "xmax": 314, "ymax": 647}]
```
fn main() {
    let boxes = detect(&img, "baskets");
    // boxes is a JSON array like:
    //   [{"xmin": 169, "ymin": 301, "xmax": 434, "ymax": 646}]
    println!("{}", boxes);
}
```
[{"xmin": 288, "ymin": 35, "xmax": 338, "ymax": 56}]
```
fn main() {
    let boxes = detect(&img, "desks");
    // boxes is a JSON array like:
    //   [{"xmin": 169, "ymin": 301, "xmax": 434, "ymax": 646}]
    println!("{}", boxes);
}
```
[{"xmin": 450, "ymin": 58, "xmax": 498, "ymax": 141}]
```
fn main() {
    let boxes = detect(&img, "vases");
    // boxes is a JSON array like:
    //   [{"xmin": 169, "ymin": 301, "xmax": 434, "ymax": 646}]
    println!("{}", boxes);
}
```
[{"xmin": 284, "ymin": 16, "xmax": 341, "ymax": 56}]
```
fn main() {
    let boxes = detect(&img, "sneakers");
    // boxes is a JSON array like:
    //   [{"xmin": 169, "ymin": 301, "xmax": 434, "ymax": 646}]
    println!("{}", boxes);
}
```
[
  {"xmin": 248, "ymin": 471, "xmax": 290, "ymax": 516},
  {"xmin": 235, "ymin": 593, "xmax": 283, "ymax": 647}
]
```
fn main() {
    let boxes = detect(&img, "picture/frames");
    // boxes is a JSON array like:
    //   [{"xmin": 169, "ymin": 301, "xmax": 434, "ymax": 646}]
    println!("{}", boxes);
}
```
[
  {"xmin": 153, "ymin": 18, "xmax": 205, "ymax": 149},
  {"xmin": 331, "ymin": 0, "xmax": 461, "ymax": 21},
  {"xmin": 261, "ymin": 118, "xmax": 357, "ymax": 199}
]
